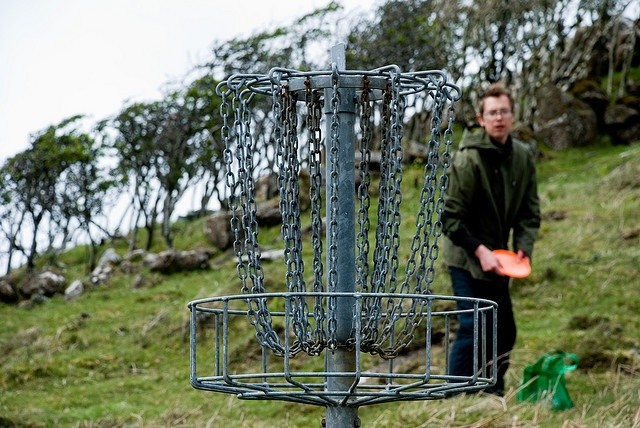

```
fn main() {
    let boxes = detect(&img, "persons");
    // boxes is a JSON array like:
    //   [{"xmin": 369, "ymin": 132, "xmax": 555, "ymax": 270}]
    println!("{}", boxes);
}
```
[{"xmin": 437, "ymin": 84, "xmax": 540, "ymax": 396}]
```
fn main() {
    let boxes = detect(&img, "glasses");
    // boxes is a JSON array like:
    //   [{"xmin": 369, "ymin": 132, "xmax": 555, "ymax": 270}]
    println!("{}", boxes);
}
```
[{"xmin": 482, "ymin": 108, "xmax": 513, "ymax": 119}]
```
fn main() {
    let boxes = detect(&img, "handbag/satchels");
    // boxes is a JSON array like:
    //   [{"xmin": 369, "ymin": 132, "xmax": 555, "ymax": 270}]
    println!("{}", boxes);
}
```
[{"xmin": 517, "ymin": 349, "xmax": 579, "ymax": 411}]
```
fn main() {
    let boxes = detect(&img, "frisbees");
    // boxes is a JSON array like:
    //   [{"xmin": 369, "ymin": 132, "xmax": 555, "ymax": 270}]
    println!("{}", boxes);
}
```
[{"xmin": 492, "ymin": 249, "xmax": 532, "ymax": 278}]
